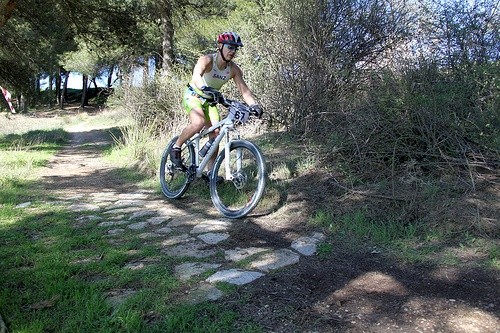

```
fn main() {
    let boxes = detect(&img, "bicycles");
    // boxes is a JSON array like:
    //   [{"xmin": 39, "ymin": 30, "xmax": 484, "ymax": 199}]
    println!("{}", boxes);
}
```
[{"xmin": 158, "ymin": 85, "xmax": 268, "ymax": 219}]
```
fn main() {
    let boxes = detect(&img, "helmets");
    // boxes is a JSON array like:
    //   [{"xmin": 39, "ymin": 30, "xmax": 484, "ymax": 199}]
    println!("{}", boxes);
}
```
[{"xmin": 217, "ymin": 31, "xmax": 244, "ymax": 47}]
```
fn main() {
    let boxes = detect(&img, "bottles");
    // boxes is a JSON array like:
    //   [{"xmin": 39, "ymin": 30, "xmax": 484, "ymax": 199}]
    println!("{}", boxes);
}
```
[{"xmin": 198, "ymin": 139, "xmax": 215, "ymax": 158}]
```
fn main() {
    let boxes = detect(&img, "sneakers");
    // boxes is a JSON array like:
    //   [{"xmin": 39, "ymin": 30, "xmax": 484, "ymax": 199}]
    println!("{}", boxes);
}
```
[
  {"xmin": 205, "ymin": 174, "xmax": 223, "ymax": 183},
  {"xmin": 169, "ymin": 142, "xmax": 182, "ymax": 166}
]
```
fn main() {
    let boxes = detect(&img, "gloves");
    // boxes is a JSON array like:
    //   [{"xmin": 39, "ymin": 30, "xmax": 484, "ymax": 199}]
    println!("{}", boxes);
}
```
[
  {"xmin": 200, "ymin": 86, "xmax": 223, "ymax": 106},
  {"xmin": 251, "ymin": 105, "xmax": 264, "ymax": 119}
]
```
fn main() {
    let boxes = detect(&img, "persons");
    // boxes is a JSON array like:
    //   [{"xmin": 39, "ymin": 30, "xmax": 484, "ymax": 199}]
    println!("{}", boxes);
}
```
[{"xmin": 167, "ymin": 31, "xmax": 264, "ymax": 186}]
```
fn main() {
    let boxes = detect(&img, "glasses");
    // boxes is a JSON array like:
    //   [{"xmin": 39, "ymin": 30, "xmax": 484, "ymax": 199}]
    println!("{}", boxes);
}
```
[{"xmin": 224, "ymin": 44, "xmax": 239, "ymax": 51}]
